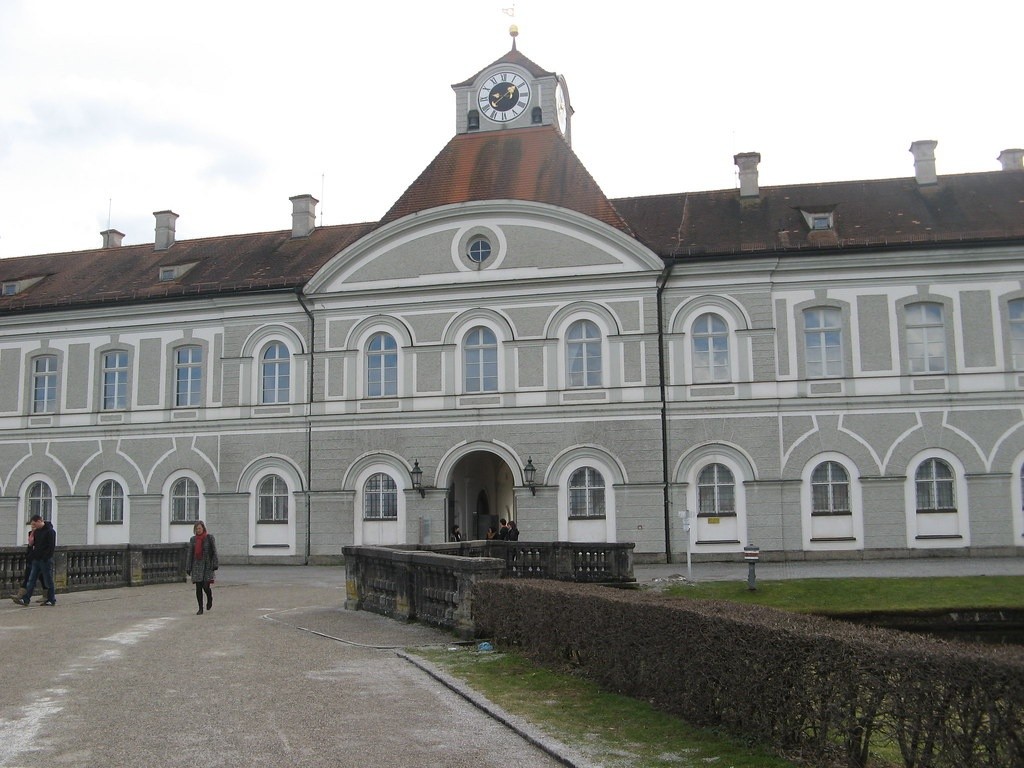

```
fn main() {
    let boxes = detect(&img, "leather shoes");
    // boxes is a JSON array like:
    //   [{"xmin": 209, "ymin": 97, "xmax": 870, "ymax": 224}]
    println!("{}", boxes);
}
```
[
  {"xmin": 18, "ymin": 597, "xmax": 28, "ymax": 606},
  {"xmin": 41, "ymin": 600, "xmax": 55, "ymax": 606}
]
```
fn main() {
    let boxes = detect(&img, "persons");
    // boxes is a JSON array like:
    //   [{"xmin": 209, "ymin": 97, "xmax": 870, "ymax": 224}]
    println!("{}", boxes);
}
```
[
  {"xmin": 11, "ymin": 516, "xmax": 56, "ymax": 606},
  {"xmin": 486, "ymin": 527, "xmax": 499, "ymax": 540},
  {"xmin": 497, "ymin": 519, "xmax": 508, "ymax": 540},
  {"xmin": 186, "ymin": 521, "xmax": 218, "ymax": 613},
  {"xmin": 450, "ymin": 525, "xmax": 462, "ymax": 542},
  {"xmin": 505, "ymin": 521, "xmax": 519, "ymax": 541}
]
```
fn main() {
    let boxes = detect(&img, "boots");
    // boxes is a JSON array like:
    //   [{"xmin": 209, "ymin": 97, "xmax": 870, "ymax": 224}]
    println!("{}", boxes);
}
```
[
  {"xmin": 36, "ymin": 590, "xmax": 48, "ymax": 603},
  {"xmin": 11, "ymin": 588, "xmax": 27, "ymax": 605},
  {"xmin": 204, "ymin": 588, "xmax": 212, "ymax": 610},
  {"xmin": 197, "ymin": 594, "xmax": 203, "ymax": 615}
]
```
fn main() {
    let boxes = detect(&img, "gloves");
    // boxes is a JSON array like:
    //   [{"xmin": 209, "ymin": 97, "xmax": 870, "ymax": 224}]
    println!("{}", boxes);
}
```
[
  {"xmin": 214, "ymin": 567, "xmax": 218, "ymax": 570},
  {"xmin": 187, "ymin": 571, "xmax": 191, "ymax": 576}
]
